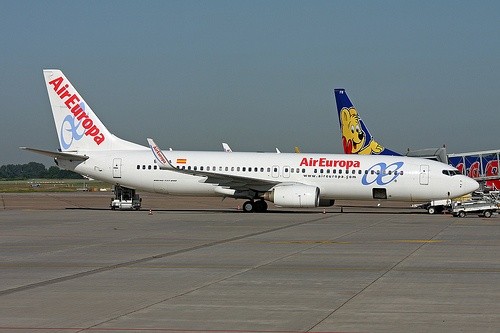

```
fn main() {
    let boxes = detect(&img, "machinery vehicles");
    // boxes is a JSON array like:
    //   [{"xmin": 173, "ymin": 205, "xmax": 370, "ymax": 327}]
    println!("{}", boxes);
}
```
[{"xmin": 410, "ymin": 192, "xmax": 500, "ymax": 217}]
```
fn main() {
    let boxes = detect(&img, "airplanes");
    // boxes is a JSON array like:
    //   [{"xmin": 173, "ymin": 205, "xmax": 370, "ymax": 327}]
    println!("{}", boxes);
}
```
[{"xmin": 19, "ymin": 69, "xmax": 479, "ymax": 215}]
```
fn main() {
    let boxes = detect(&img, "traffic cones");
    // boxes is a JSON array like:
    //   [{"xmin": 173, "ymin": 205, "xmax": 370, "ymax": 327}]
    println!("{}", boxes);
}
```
[
  {"xmin": 148, "ymin": 208, "xmax": 152, "ymax": 215},
  {"xmin": 323, "ymin": 208, "xmax": 326, "ymax": 214},
  {"xmin": 376, "ymin": 201, "xmax": 380, "ymax": 207},
  {"xmin": 237, "ymin": 203, "xmax": 241, "ymax": 210}
]
